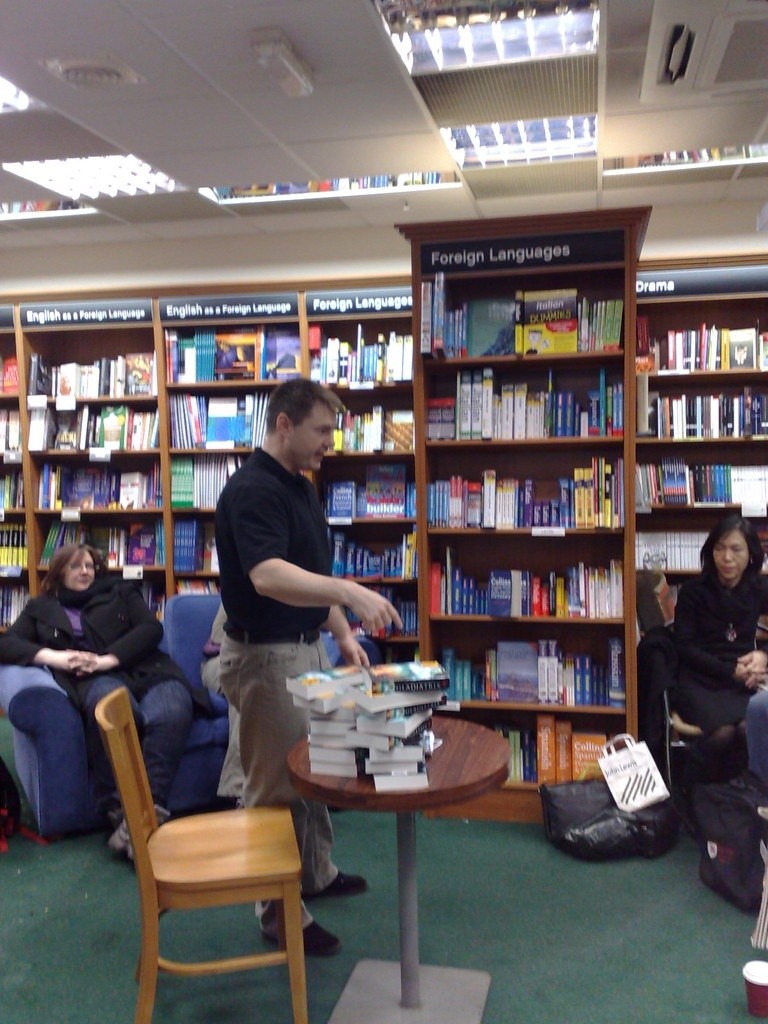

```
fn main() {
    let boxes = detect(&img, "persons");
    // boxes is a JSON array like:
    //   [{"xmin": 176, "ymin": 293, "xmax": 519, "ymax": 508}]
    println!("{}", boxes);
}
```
[
  {"xmin": 200, "ymin": 602, "xmax": 247, "ymax": 799},
  {"xmin": 0, "ymin": 543, "xmax": 216, "ymax": 860},
  {"xmin": 671, "ymin": 514, "xmax": 768, "ymax": 750},
  {"xmin": 214, "ymin": 378, "xmax": 404, "ymax": 954}
]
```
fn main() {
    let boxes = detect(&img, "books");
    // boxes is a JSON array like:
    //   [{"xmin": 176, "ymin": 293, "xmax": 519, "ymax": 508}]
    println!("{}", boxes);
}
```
[
  {"xmin": 0, "ymin": 326, "xmax": 417, "ymax": 640},
  {"xmin": 285, "ymin": 660, "xmax": 451, "ymax": 792},
  {"xmin": 420, "ymin": 271, "xmax": 768, "ymax": 784}
]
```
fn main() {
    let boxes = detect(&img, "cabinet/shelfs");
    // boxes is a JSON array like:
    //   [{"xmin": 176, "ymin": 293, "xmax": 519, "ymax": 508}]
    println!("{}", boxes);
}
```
[
  {"xmin": 0, "ymin": 304, "xmax": 30, "ymax": 641},
  {"xmin": 155, "ymin": 294, "xmax": 305, "ymax": 596},
  {"xmin": 394, "ymin": 204, "xmax": 652, "ymax": 789},
  {"xmin": 632, "ymin": 251, "xmax": 767, "ymax": 641},
  {"xmin": 303, "ymin": 288, "xmax": 419, "ymax": 660},
  {"xmin": 15, "ymin": 300, "xmax": 168, "ymax": 620}
]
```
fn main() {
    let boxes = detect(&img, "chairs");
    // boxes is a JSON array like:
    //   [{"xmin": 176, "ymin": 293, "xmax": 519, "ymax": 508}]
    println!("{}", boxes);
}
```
[
  {"xmin": 96, "ymin": 686, "xmax": 308, "ymax": 1024},
  {"xmin": 661, "ymin": 621, "xmax": 703, "ymax": 789}
]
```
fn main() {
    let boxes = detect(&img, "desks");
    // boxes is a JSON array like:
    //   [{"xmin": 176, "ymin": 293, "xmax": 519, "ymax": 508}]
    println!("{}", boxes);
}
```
[{"xmin": 287, "ymin": 716, "xmax": 512, "ymax": 1024}]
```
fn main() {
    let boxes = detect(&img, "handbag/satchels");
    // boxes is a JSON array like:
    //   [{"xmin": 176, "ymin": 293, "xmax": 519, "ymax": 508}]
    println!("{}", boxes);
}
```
[
  {"xmin": 692, "ymin": 770, "xmax": 768, "ymax": 910},
  {"xmin": 596, "ymin": 734, "xmax": 670, "ymax": 813},
  {"xmin": 537, "ymin": 780, "xmax": 612, "ymax": 842}
]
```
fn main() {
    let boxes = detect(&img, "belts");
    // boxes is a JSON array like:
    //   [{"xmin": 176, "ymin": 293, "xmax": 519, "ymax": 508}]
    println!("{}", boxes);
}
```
[{"xmin": 225, "ymin": 626, "xmax": 317, "ymax": 643}]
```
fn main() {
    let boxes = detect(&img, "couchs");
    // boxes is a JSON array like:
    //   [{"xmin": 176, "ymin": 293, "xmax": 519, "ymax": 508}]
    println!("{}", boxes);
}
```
[{"xmin": 0, "ymin": 593, "xmax": 385, "ymax": 841}]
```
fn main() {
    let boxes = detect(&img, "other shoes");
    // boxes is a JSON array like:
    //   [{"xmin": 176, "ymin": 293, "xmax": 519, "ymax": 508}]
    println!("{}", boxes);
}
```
[
  {"xmin": 262, "ymin": 919, "xmax": 342, "ymax": 958},
  {"xmin": 300, "ymin": 871, "xmax": 369, "ymax": 900},
  {"xmin": 108, "ymin": 804, "xmax": 173, "ymax": 866}
]
